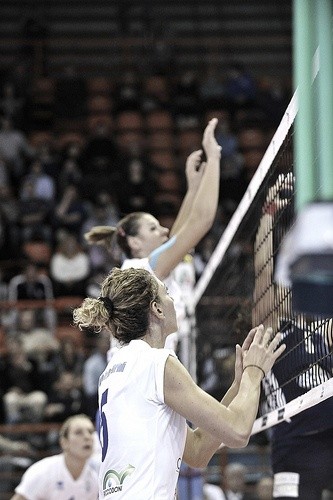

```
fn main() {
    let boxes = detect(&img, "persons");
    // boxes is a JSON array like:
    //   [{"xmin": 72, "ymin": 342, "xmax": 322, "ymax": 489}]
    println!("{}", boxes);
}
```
[
  {"xmin": 52, "ymin": 335, "xmax": 85, "ymax": 407},
  {"xmin": 251, "ymin": 171, "xmax": 333, "ymax": 499},
  {"xmin": 10, "ymin": 309, "xmax": 59, "ymax": 348},
  {"xmin": 73, "ymin": 266, "xmax": 285, "ymax": 500},
  {"xmin": 8, "ymin": 262, "xmax": 55, "ymax": 305},
  {"xmin": 4, "ymin": 336, "xmax": 47, "ymax": 424},
  {"xmin": 85, "ymin": 117, "xmax": 223, "ymax": 354},
  {"xmin": 2, "ymin": 70, "xmax": 291, "ymax": 289},
  {"xmin": 80, "ymin": 328, "xmax": 112, "ymax": 413},
  {"xmin": 11, "ymin": 414, "xmax": 103, "ymax": 500},
  {"xmin": 214, "ymin": 462, "xmax": 255, "ymax": 500},
  {"xmin": 253, "ymin": 472, "xmax": 276, "ymax": 500}
]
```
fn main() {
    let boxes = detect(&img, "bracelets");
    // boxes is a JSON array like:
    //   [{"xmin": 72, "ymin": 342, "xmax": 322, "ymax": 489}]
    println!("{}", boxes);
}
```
[
  {"xmin": 261, "ymin": 199, "xmax": 278, "ymax": 216},
  {"xmin": 241, "ymin": 364, "xmax": 266, "ymax": 378}
]
